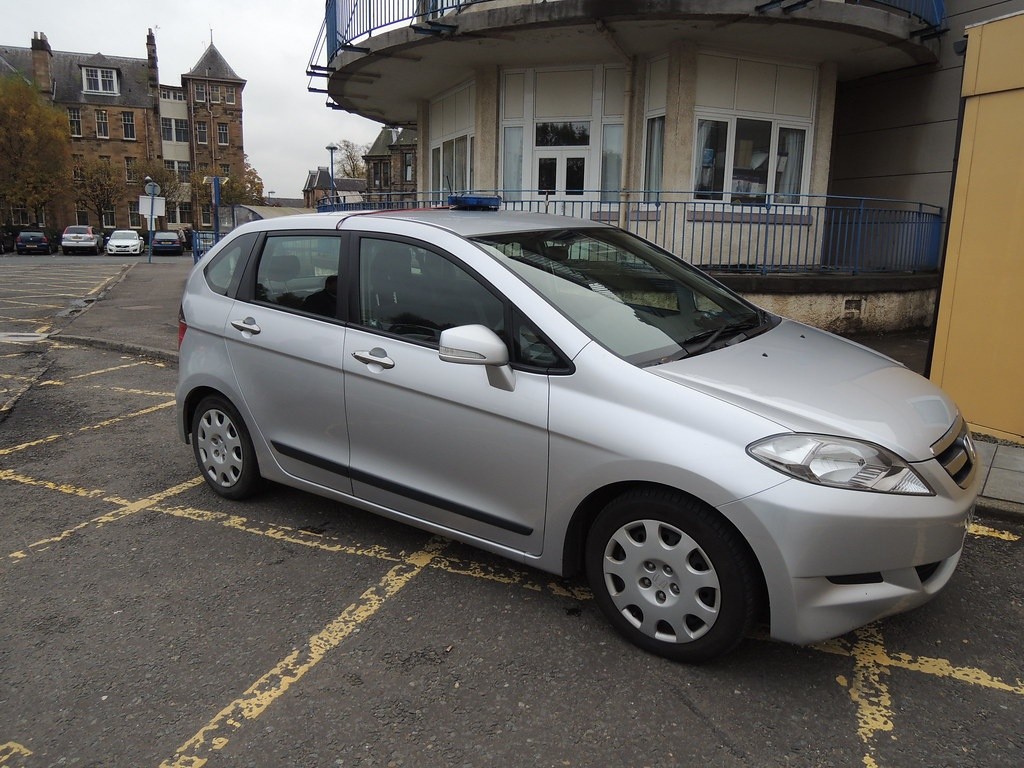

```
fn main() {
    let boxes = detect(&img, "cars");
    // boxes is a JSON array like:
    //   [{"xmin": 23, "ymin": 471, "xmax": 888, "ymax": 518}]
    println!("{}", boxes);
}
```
[
  {"xmin": 151, "ymin": 231, "xmax": 183, "ymax": 255},
  {"xmin": 173, "ymin": 195, "xmax": 982, "ymax": 666},
  {"xmin": 15, "ymin": 231, "xmax": 58, "ymax": 255},
  {"xmin": 105, "ymin": 229, "xmax": 145, "ymax": 255}
]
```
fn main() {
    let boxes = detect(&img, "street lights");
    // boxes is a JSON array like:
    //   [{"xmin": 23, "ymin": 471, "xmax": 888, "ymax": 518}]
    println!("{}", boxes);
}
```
[
  {"xmin": 268, "ymin": 191, "xmax": 275, "ymax": 198},
  {"xmin": 145, "ymin": 175, "xmax": 154, "ymax": 264},
  {"xmin": 326, "ymin": 142, "xmax": 339, "ymax": 211}
]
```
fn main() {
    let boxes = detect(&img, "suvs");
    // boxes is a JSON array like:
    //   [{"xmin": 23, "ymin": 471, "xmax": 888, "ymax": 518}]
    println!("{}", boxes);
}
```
[{"xmin": 61, "ymin": 224, "xmax": 104, "ymax": 255}]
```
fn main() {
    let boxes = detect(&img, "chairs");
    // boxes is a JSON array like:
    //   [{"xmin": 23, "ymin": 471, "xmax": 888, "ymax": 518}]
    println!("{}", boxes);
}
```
[{"xmin": 371, "ymin": 248, "xmax": 443, "ymax": 342}]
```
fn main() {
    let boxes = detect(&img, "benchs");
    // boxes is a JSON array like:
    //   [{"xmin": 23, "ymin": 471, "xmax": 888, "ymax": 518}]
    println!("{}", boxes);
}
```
[{"xmin": 263, "ymin": 255, "xmax": 338, "ymax": 316}]
